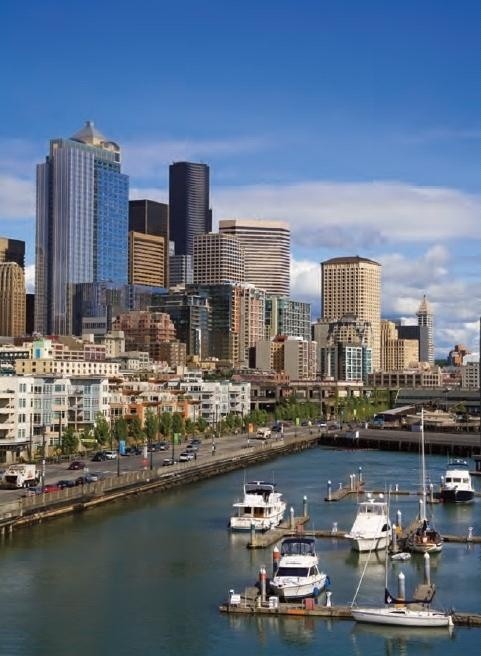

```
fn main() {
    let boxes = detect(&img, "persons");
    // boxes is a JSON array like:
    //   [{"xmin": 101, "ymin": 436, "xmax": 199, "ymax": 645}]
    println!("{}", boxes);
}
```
[{"xmin": 211, "ymin": 442, "xmax": 216, "ymax": 457}]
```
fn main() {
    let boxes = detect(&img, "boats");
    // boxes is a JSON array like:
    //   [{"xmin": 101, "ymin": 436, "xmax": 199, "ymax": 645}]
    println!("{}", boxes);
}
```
[
  {"xmin": 227, "ymin": 531, "xmax": 264, "ymax": 555},
  {"xmin": 440, "ymin": 498, "xmax": 480, "ymax": 524},
  {"xmin": 220, "ymin": 611, "xmax": 459, "ymax": 656},
  {"xmin": 346, "ymin": 547, "xmax": 442, "ymax": 580}
]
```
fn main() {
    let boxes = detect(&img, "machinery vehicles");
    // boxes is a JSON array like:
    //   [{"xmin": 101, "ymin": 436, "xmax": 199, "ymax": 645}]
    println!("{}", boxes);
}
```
[{"xmin": 2, "ymin": 463, "xmax": 42, "ymax": 489}]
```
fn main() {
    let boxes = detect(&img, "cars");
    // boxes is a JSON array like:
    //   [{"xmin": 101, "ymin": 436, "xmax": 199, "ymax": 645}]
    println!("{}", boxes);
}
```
[
  {"xmin": 256, "ymin": 420, "xmax": 327, "ymax": 439},
  {"xmin": 24, "ymin": 438, "xmax": 202, "ymax": 498}
]
